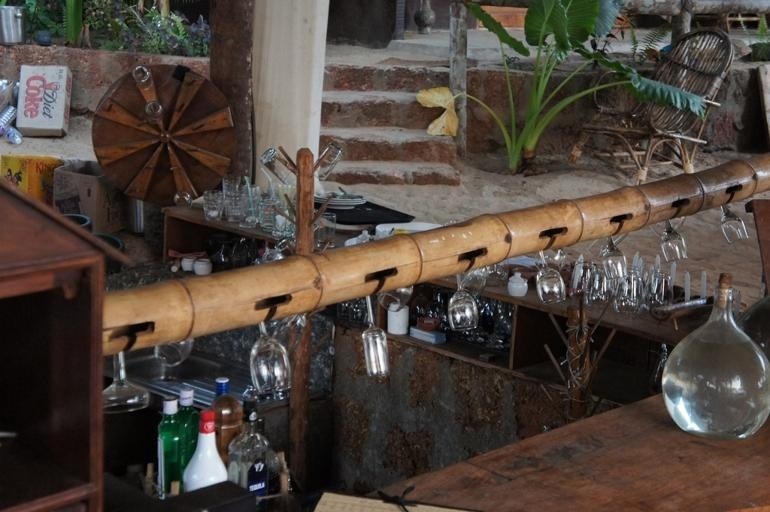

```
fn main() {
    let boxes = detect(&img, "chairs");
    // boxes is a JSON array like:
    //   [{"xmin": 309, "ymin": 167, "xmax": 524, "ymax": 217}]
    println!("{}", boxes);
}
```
[{"xmin": 566, "ymin": 25, "xmax": 735, "ymax": 188}]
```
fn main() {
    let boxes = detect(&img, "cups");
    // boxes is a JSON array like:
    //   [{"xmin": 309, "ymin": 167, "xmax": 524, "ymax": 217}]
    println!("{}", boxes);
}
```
[
  {"xmin": 153, "ymin": 338, "xmax": 196, "ymax": 368},
  {"xmin": 313, "ymin": 141, "xmax": 343, "ymax": 180},
  {"xmin": 335, "ymin": 301, "xmax": 367, "ymax": 322},
  {"xmin": 201, "ymin": 175, "xmax": 338, "ymax": 249},
  {"xmin": 256, "ymin": 147, "xmax": 298, "ymax": 190},
  {"xmin": 575, "ymin": 264, "xmax": 672, "ymax": 318}
]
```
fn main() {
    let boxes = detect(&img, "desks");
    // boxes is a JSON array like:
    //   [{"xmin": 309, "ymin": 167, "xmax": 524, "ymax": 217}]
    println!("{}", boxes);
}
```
[{"xmin": 359, "ymin": 393, "xmax": 770, "ymax": 510}]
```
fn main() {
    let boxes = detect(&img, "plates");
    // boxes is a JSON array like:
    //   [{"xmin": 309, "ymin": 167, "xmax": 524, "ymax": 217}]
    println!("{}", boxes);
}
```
[{"xmin": 312, "ymin": 191, "xmax": 367, "ymax": 211}]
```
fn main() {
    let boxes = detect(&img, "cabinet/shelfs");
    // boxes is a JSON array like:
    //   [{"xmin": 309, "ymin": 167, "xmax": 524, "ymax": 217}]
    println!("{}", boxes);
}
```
[
  {"xmin": 162, "ymin": 199, "xmax": 735, "ymax": 412},
  {"xmin": 1, "ymin": 181, "xmax": 132, "ymax": 512}
]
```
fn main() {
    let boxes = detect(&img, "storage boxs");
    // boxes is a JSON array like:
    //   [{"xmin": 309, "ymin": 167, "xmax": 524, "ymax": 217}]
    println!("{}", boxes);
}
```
[
  {"xmin": 15, "ymin": 62, "xmax": 73, "ymax": 138},
  {"xmin": 1, "ymin": 154, "xmax": 124, "ymax": 235}
]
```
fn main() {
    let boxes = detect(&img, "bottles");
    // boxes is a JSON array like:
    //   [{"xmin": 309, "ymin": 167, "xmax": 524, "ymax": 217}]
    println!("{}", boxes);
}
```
[
  {"xmin": 153, "ymin": 375, "xmax": 277, "ymax": 500},
  {"xmin": 181, "ymin": 255, "xmax": 213, "ymax": 276},
  {"xmin": 0, "ymin": 104, "xmax": 24, "ymax": 146},
  {"xmin": 661, "ymin": 270, "xmax": 767, "ymax": 443}
]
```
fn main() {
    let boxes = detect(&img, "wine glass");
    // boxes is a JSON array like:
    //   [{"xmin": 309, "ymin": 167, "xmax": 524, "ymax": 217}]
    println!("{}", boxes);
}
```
[
  {"xmin": 533, "ymin": 245, "xmax": 568, "ymax": 307},
  {"xmin": 601, "ymin": 228, "xmax": 629, "ymax": 281},
  {"xmin": 101, "ymin": 350, "xmax": 151, "ymax": 416},
  {"xmin": 719, "ymin": 201, "xmax": 749, "ymax": 245},
  {"xmin": 447, "ymin": 270, "xmax": 480, "ymax": 332},
  {"xmin": 659, "ymin": 214, "xmax": 688, "ymax": 265},
  {"xmin": 249, "ymin": 318, "xmax": 294, "ymax": 398},
  {"xmin": 360, "ymin": 292, "xmax": 390, "ymax": 381}
]
